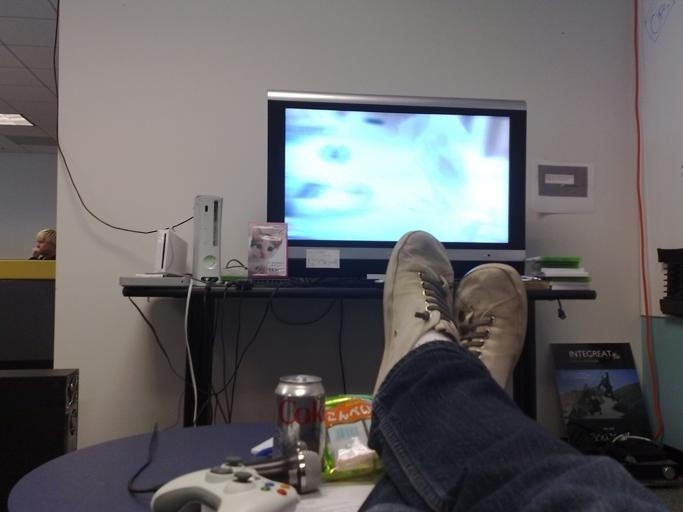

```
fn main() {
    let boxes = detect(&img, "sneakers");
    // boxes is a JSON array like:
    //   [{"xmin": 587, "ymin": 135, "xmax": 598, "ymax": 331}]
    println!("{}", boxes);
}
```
[{"xmin": 374, "ymin": 230, "xmax": 529, "ymax": 403}]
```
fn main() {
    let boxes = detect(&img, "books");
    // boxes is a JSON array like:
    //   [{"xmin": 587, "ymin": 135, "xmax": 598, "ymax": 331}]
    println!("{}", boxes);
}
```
[
  {"xmin": 527, "ymin": 256, "xmax": 592, "ymax": 291},
  {"xmin": 551, "ymin": 343, "xmax": 653, "ymax": 446}
]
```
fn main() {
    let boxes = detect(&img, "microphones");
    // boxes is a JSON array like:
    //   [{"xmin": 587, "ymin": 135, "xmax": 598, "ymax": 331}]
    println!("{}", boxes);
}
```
[{"xmin": 248, "ymin": 449, "xmax": 321, "ymax": 494}]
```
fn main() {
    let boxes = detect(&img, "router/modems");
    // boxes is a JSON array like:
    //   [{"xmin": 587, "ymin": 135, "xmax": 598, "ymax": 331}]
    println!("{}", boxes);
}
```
[{"xmin": 155, "ymin": 229, "xmax": 188, "ymax": 277}]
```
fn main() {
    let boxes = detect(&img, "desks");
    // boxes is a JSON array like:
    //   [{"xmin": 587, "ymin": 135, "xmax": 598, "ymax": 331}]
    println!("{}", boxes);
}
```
[
  {"xmin": 7, "ymin": 424, "xmax": 280, "ymax": 510},
  {"xmin": 122, "ymin": 285, "xmax": 599, "ymax": 426}
]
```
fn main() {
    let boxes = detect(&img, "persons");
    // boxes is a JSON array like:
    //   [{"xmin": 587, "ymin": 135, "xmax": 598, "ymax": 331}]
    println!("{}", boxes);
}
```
[
  {"xmin": 28, "ymin": 229, "xmax": 56, "ymax": 260},
  {"xmin": 356, "ymin": 231, "xmax": 673, "ymax": 512}
]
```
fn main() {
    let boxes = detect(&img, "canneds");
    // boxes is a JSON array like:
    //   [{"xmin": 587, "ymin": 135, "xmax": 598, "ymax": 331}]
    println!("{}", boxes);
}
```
[{"xmin": 270, "ymin": 373, "xmax": 327, "ymax": 495}]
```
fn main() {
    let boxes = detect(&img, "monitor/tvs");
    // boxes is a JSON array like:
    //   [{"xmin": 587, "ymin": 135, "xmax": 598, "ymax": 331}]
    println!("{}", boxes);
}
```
[{"xmin": 265, "ymin": 91, "xmax": 527, "ymax": 287}]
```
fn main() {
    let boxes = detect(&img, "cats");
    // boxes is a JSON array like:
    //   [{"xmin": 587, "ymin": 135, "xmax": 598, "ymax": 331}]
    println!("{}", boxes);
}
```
[{"xmin": 249, "ymin": 227, "xmax": 284, "ymax": 275}]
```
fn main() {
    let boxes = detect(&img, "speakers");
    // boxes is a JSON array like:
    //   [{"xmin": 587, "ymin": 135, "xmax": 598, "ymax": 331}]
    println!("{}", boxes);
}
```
[
  {"xmin": 0, "ymin": 368, "xmax": 79, "ymax": 512},
  {"xmin": 193, "ymin": 194, "xmax": 223, "ymax": 286}
]
